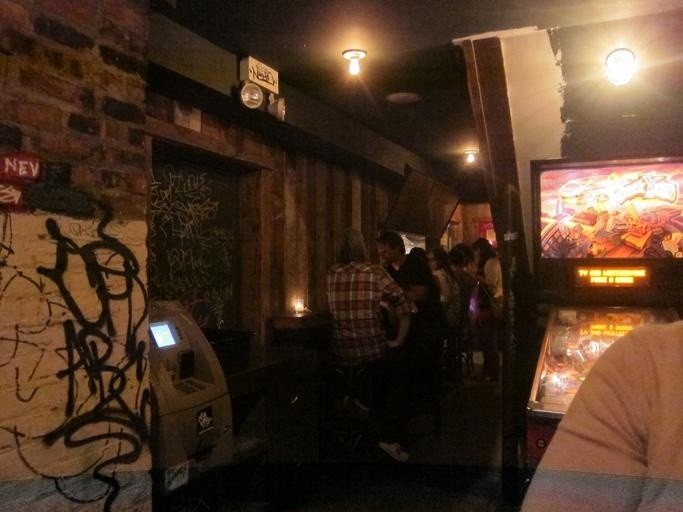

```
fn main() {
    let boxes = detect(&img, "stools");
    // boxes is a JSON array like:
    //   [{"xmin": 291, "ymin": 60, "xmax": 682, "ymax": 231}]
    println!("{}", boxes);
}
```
[{"xmin": 324, "ymin": 312, "xmax": 471, "ymax": 435}]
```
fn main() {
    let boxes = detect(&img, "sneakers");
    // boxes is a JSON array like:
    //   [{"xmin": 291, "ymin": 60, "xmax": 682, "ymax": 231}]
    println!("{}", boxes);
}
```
[{"xmin": 376, "ymin": 442, "xmax": 409, "ymax": 462}]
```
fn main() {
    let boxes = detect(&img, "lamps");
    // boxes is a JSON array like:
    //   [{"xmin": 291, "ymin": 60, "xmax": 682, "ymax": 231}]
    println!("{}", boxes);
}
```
[
  {"xmin": 237, "ymin": 80, "xmax": 287, "ymax": 123},
  {"xmin": 343, "ymin": 49, "xmax": 368, "ymax": 76}
]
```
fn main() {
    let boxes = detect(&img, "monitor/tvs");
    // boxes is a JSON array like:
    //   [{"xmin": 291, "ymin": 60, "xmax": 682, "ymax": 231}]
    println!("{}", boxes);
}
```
[{"xmin": 529, "ymin": 152, "xmax": 682, "ymax": 305}]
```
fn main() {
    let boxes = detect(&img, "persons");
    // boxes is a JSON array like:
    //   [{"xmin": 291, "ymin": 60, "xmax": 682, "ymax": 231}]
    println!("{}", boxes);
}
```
[
  {"xmin": 520, "ymin": 317, "xmax": 682, "ymax": 510},
  {"xmin": 328, "ymin": 227, "xmax": 503, "ymax": 461}
]
